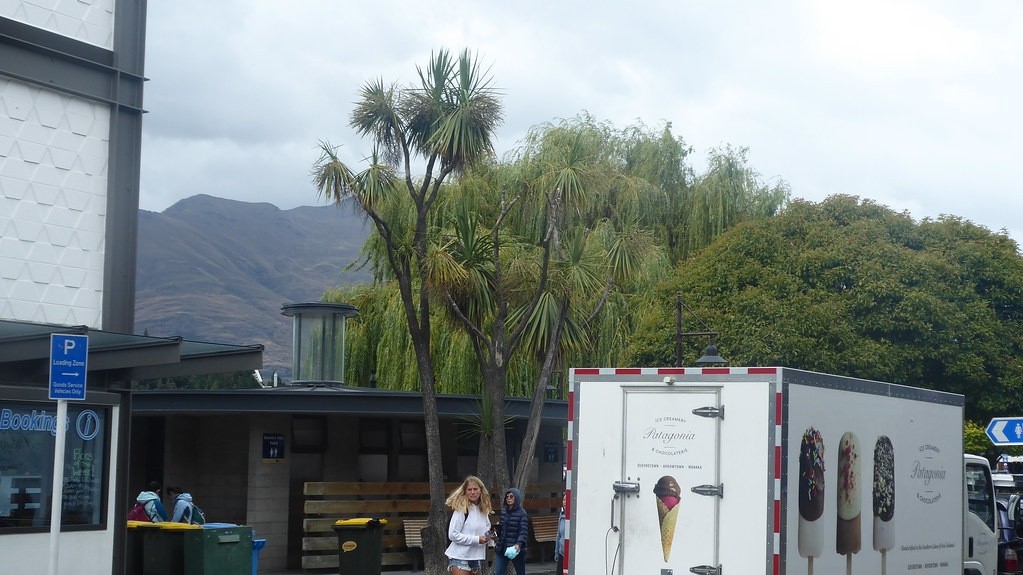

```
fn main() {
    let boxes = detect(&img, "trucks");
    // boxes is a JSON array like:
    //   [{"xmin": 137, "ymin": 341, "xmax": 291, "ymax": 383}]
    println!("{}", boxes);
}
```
[{"xmin": 560, "ymin": 364, "xmax": 1020, "ymax": 575}]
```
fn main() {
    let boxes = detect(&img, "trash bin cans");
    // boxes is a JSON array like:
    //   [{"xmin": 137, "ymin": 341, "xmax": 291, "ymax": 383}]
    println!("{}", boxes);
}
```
[
  {"xmin": 332, "ymin": 517, "xmax": 388, "ymax": 575},
  {"xmin": 203, "ymin": 522, "xmax": 266, "ymax": 575},
  {"xmin": 127, "ymin": 520, "xmax": 204, "ymax": 575}
]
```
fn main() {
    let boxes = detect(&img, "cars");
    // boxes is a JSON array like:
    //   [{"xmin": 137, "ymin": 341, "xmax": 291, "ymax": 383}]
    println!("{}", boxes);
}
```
[{"xmin": 968, "ymin": 495, "xmax": 1023, "ymax": 575}]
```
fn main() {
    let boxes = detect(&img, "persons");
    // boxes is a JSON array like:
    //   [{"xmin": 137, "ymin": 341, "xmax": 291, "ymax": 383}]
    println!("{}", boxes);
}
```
[
  {"xmin": 136, "ymin": 481, "xmax": 169, "ymax": 522},
  {"xmin": 444, "ymin": 476, "xmax": 492, "ymax": 575},
  {"xmin": 554, "ymin": 492, "xmax": 566, "ymax": 575},
  {"xmin": 494, "ymin": 488, "xmax": 529, "ymax": 575},
  {"xmin": 166, "ymin": 486, "xmax": 193, "ymax": 523}
]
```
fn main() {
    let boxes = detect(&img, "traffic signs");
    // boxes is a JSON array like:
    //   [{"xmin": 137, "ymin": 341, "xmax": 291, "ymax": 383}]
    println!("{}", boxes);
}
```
[
  {"xmin": 984, "ymin": 417, "xmax": 1023, "ymax": 446},
  {"xmin": 49, "ymin": 334, "xmax": 87, "ymax": 400}
]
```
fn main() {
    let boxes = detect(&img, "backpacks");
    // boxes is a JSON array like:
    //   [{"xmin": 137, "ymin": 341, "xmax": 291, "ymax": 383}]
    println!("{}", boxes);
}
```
[
  {"xmin": 127, "ymin": 499, "xmax": 156, "ymax": 522},
  {"xmin": 184, "ymin": 502, "xmax": 205, "ymax": 524}
]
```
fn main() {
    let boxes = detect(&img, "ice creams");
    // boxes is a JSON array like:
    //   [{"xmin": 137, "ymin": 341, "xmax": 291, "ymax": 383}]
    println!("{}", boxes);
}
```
[{"xmin": 653, "ymin": 476, "xmax": 681, "ymax": 563}]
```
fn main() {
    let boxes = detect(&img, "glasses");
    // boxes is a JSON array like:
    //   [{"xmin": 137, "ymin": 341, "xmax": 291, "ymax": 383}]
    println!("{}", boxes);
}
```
[{"xmin": 505, "ymin": 495, "xmax": 514, "ymax": 499}]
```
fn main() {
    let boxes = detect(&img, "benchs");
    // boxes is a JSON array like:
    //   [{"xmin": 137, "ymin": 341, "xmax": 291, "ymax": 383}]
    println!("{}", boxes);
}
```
[
  {"xmin": 530, "ymin": 516, "xmax": 561, "ymax": 563},
  {"xmin": 403, "ymin": 520, "xmax": 431, "ymax": 572}
]
